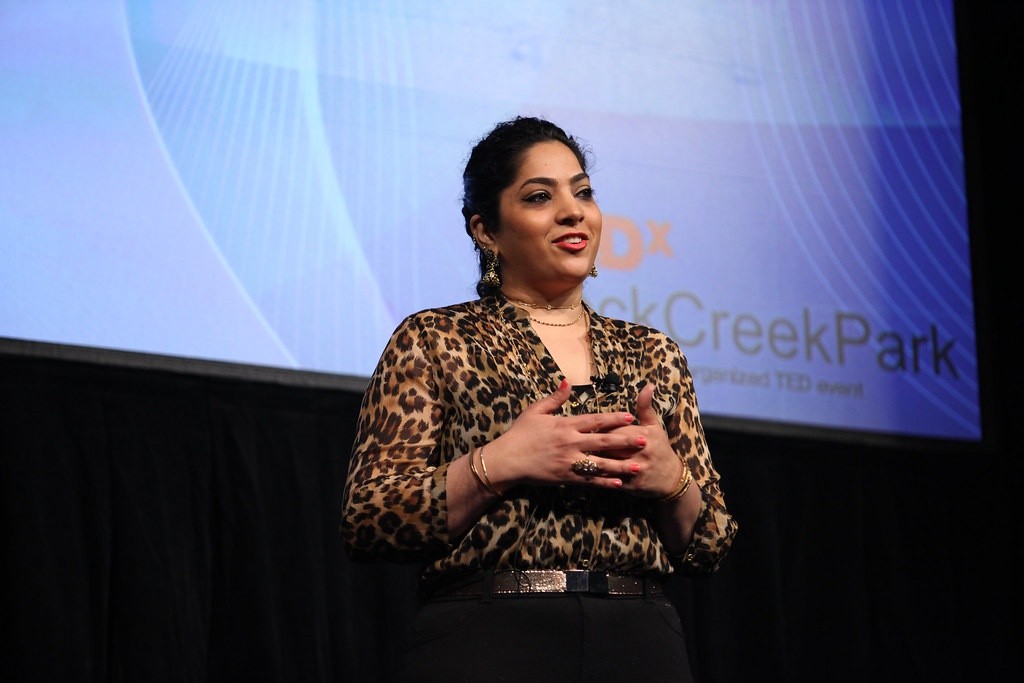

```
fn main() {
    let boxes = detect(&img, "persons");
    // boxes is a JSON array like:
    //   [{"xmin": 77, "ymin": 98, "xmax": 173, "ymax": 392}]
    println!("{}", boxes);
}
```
[{"xmin": 339, "ymin": 117, "xmax": 739, "ymax": 683}]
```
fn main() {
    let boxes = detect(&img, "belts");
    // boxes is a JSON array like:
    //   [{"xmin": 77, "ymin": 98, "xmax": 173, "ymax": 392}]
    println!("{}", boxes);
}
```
[{"xmin": 414, "ymin": 569, "xmax": 666, "ymax": 599}]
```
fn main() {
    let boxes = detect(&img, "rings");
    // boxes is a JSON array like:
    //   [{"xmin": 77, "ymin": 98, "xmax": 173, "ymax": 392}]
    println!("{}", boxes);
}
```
[{"xmin": 572, "ymin": 452, "xmax": 599, "ymax": 476}]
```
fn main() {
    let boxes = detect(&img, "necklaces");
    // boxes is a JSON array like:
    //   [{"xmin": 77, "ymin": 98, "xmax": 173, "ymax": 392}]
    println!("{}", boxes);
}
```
[
  {"xmin": 584, "ymin": 310, "xmax": 600, "ymax": 413},
  {"xmin": 529, "ymin": 305, "xmax": 585, "ymax": 326},
  {"xmin": 502, "ymin": 294, "xmax": 582, "ymax": 309}
]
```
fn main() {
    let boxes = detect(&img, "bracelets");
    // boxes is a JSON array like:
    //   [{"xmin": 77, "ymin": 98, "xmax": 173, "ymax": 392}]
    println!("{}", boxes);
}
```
[
  {"xmin": 469, "ymin": 444, "xmax": 501, "ymax": 498},
  {"xmin": 642, "ymin": 454, "xmax": 692, "ymax": 505}
]
